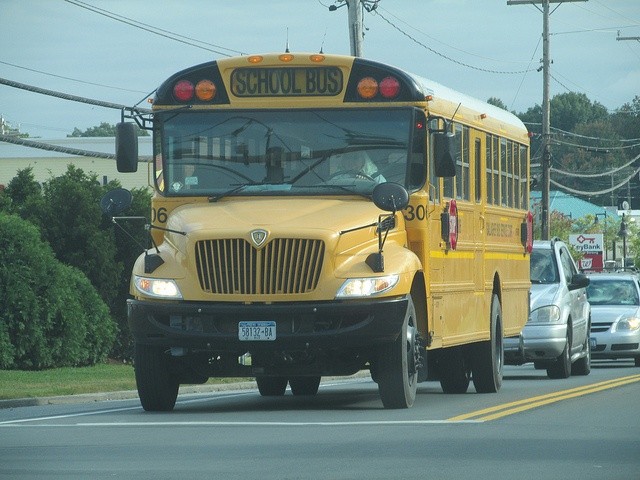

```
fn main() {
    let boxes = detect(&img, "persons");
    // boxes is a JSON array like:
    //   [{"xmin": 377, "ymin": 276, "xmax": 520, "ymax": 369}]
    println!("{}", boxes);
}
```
[{"xmin": 330, "ymin": 144, "xmax": 388, "ymax": 184}]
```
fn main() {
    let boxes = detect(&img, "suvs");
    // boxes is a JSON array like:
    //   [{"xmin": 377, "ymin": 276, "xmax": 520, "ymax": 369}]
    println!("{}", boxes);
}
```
[{"xmin": 503, "ymin": 236, "xmax": 591, "ymax": 378}]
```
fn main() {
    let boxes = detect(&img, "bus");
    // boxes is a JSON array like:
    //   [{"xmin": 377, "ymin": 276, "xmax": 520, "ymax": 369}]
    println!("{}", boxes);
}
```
[{"xmin": 115, "ymin": 53, "xmax": 533, "ymax": 411}]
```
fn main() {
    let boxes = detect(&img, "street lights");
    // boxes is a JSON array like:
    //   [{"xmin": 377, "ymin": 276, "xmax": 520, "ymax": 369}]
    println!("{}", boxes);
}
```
[
  {"xmin": 593, "ymin": 211, "xmax": 607, "ymax": 260},
  {"xmin": 617, "ymin": 213, "xmax": 628, "ymax": 271}
]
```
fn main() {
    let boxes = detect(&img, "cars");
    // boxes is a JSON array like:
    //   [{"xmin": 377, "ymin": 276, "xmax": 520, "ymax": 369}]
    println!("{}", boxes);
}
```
[{"xmin": 584, "ymin": 274, "xmax": 640, "ymax": 366}]
